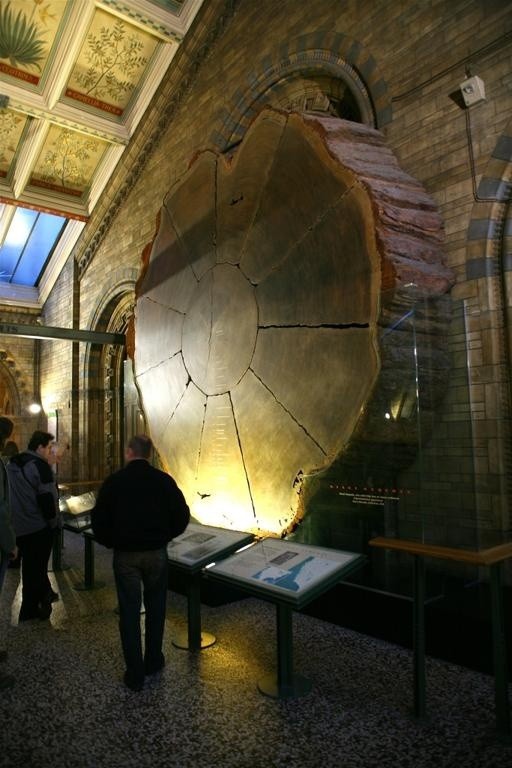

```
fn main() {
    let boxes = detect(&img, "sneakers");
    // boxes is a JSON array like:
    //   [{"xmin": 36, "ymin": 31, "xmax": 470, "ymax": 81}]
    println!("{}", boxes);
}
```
[
  {"xmin": 124, "ymin": 649, "xmax": 165, "ymax": 694},
  {"xmin": 1, "ymin": 650, "xmax": 12, "ymax": 664}
]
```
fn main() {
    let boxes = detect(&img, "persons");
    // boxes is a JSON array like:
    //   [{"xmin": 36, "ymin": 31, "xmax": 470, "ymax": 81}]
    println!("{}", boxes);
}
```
[
  {"xmin": 1, "ymin": 415, "xmax": 20, "ymax": 697},
  {"xmin": 1, "ymin": 440, "xmax": 20, "ymax": 471},
  {"xmin": 90, "ymin": 432, "xmax": 191, "ymax": 692},
  {"xmin": 6, "ymin": 430, "xmax": 67, "ymax": 623}
]
